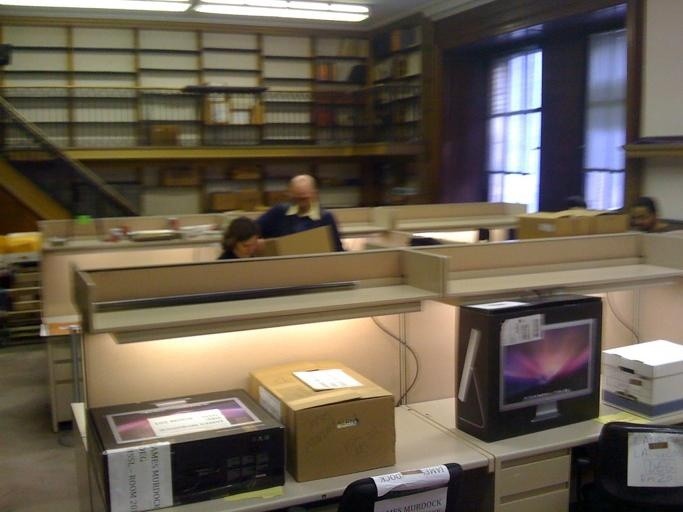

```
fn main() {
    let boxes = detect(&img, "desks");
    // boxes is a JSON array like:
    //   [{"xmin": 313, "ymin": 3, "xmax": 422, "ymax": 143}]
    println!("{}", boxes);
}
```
[
  {"xmin": 39, "ymin": 206, "xmax": 380, "ymax": 337},
  {"xmin": 404, "ymin": 230, "xmax": 683, "ymax": 465},
  {"xmin": 64, "ymin": 248, "xmax": 490, "ymax": 511}
]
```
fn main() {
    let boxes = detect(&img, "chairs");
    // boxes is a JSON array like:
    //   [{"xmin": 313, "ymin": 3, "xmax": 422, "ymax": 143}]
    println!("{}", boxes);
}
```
[
  {"xmin": 339, "ymin": 461, "xmax": 463, "ymax": 511},
  {"xmin": 572, "ymin": 420, "xmax": 683, "ymax": 511}
]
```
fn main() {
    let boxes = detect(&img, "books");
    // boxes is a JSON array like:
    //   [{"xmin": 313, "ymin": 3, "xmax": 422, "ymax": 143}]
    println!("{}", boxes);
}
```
[{"xmin": 312, "ymin": 22, "xmax": 424, "ymax": 146}]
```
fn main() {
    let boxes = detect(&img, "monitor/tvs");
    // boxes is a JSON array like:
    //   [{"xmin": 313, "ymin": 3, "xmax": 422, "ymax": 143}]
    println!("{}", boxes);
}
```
[
  {"xmin": 498, "ymin": 318, "xmax": 595, "ymax": 424},
  {"xmin": 105, "ymin": 397, "xmax": 263, "ymax": 445}
]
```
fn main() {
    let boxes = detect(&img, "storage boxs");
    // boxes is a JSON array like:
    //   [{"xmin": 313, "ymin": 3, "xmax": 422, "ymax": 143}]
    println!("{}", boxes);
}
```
[
  {"xmin": 1, "ymin": 231, "xmax": 44, "ymax": 264},
  {"xmin": 455, "ymin": 295, "xmax": 603, "ymax": 443},
  {"xmin": 248, "ymin": 358, "xmax": 397, "ymax": 483},
  {"xmin": 200, "ymin": 92, "xmax": 267, "ymax": 126},
  {"xmin": 83, "ymin": 388, "xmax": 285, "ymax": 512},
  {"xmin": 258, "ymin": 225, "xmax": 333, "ymax": 255},
  {"xmin": 142, "ymin": 124, "xmax": 183, "ymax": 146},
  {"xmin": 515, "ymin": 208, "xmax": 633, "ymax": 240},
  {"xmin": 139, "ymin": 162, "xmax": 432, "ymax": 211},
  {"xmin": 598, "ymin": 338, "xmax": 683, "ymax": 420}
]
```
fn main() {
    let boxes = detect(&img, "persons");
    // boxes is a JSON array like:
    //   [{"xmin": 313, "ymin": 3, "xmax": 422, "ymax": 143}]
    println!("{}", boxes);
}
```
[
  {"xmin": 627, "ymin": 196, "xmax": 683, "ymax": 236},
  {"xmin": 567, "ymin": 195, "xmax": 586, "ymax": 208},
  {"xmin": 215, "ymin": 215, "xmax": 264, "ymax": 260},
  {"xmin": 252, "ymin": 173, "xmax": 345, "ymax": 256}
]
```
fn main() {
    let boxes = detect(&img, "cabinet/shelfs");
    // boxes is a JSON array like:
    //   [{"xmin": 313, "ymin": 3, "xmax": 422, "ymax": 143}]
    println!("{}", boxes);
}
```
[
  {"xmin": 0, "ymin": 260, "xmax": 46, "ymax": 349},
  {"xmin": 492, "ymin": 446, "xmax": 574, "ymax": 511},
  {"xmin": 47, "ymin": 338, "xmax": 84, "ymax": 435},
  {"xmin": 1, "ymin": 18, "xmax": 434, "ymax": 158}
]
